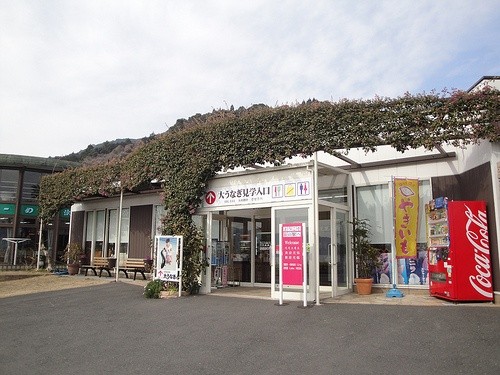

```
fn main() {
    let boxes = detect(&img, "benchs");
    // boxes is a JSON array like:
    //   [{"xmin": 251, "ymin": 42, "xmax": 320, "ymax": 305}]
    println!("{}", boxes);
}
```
[
  {"xmin": 119, "ymin": 259, "xmax": 147, "ymax": 281},
  {"xmin": 83, "ymin": 257, "xmax": 112, "ymax": 277}
]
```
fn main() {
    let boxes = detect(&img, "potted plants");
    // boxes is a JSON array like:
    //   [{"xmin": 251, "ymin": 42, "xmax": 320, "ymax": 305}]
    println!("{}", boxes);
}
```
[
  {"xmin": 60, "ymin": 242, "xmax": 84, "ymax": 275},
  {"xmin": 348, "ymin": 217, "xmax": 383, "ymax": 296}
]
